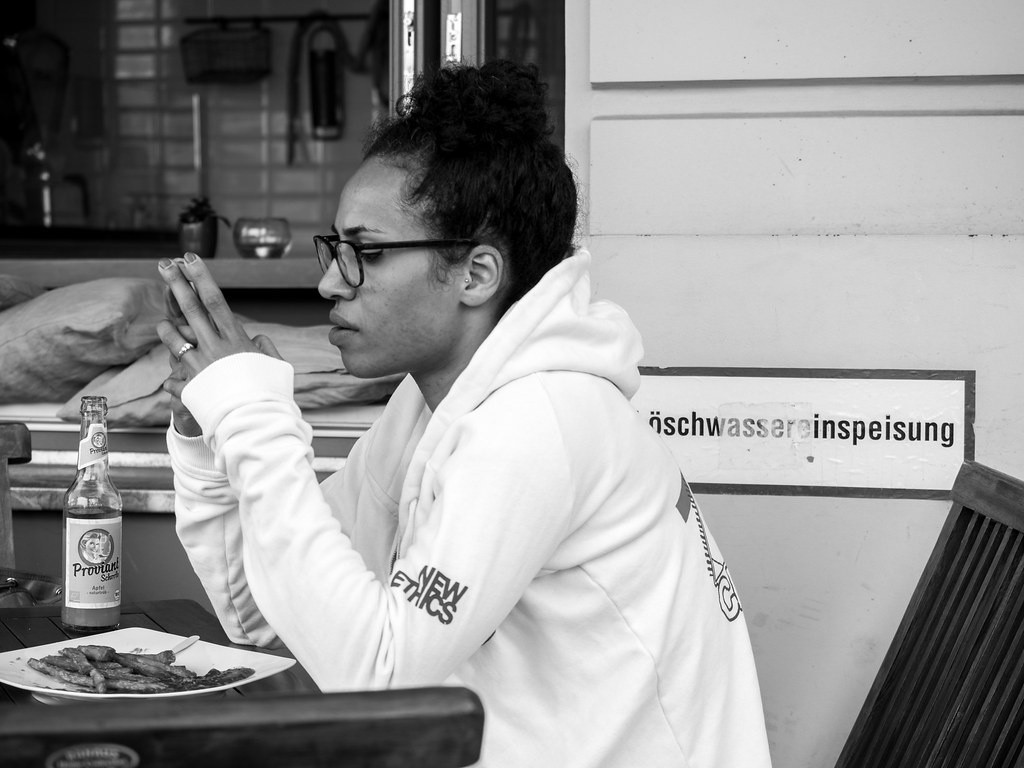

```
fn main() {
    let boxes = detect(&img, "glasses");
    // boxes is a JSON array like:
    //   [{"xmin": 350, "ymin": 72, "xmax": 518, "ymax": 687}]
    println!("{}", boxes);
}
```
[{"xmin": 313, "ymin": 235, "xmax": 481, "ymax": 288}]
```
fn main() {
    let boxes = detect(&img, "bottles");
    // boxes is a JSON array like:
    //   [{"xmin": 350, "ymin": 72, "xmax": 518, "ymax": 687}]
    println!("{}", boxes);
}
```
[{"xmin": 60, "ymin": 396, "xmax": 123, "ymax": 634}]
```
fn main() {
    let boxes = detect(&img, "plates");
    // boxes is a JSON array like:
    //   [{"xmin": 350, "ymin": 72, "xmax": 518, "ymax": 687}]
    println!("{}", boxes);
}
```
[{"xmin": 0, "ymin": 627, "xmax": 296, "ymax": 706}]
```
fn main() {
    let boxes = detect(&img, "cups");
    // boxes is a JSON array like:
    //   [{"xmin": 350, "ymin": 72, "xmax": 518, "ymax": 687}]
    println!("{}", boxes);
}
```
[{"xmin": 234, "ymin": 217, "xmax": 292, "ymax": 258}]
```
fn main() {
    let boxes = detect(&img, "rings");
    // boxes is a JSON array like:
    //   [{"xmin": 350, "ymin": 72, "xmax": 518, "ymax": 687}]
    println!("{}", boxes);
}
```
[{"xmin": 178, "ymin": 342, "xmax": 193, "ymax": 362}]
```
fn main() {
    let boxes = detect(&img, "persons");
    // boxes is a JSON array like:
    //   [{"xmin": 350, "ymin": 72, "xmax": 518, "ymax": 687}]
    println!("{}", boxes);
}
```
[{"xmin": 158, "ymin": 59, "xmax": 774, "ymax": 768}]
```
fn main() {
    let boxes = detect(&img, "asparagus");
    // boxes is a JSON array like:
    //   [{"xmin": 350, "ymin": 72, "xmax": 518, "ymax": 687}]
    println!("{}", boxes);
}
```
[{"xmin": 28, "ymin": 644, "xmax": 191, "ymax": 694}]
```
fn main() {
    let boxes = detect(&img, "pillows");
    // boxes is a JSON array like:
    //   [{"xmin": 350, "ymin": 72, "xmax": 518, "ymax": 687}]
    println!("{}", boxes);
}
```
[{"xmin": 1, "ymin": 275, "xmax": 407, "ymax": 425}]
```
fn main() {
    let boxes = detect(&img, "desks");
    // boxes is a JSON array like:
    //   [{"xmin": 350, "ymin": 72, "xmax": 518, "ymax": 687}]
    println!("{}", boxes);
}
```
[{"xmin": 1, "ymin": 597, "xmax": 319, "ymax": 715}]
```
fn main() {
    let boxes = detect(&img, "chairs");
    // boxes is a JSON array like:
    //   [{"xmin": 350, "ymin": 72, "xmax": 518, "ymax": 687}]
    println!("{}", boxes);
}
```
[{"xmin": 834, "ymin": 455, "xmax": 1024, "ymax": 768}]
